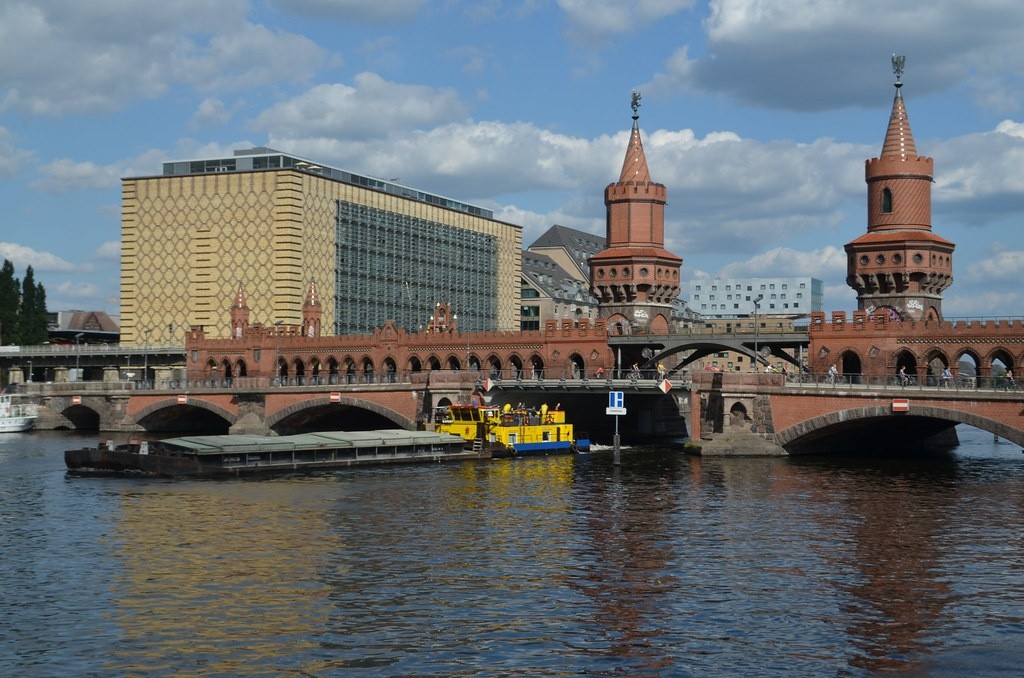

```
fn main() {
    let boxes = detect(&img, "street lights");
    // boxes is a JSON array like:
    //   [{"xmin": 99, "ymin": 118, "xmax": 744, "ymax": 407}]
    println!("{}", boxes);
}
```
[
  {"xmin": 144, "ymin": 328, "xmax": 154, "ymax": 390},
  {"xmin": 753, "ymin": 296, "xmax": 764, "ymax": 371},
  {"xmin": 273, "ymin": 319, "xmax": 284, "ymax": 387},
  {"xmin": 74, "ymin": 331, "xmax": 85, "ymax": 383},
  {"xmin": 467, "ymin": 307, "xmax": 474, "ymax": 373}
]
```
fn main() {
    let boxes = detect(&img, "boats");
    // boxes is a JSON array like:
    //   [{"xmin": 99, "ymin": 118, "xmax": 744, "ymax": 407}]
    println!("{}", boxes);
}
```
[
  {"xmin": 434, "ymin": 399, "xmax": 590, "ymax": 460},
  {"xmin": 0, "ymin": 395, "xmax": 39, "ymax": 432}
]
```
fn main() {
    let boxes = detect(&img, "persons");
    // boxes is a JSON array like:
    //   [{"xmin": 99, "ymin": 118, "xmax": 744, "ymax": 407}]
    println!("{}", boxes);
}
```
[
  {"xmin": 782, "ymin": 363, "xmax": 790, "ymax": 381},
  {"xmin": 657, "ymin": 361, "xmax": 667, "ymax": 379},
  {"xmin": 598, "ymin": 366, "xmax": 604, "ymax": 379},
  {"xmin": 828, "ymin": 364, "xmax": 838, "ymax": 385},
  {"xmin": 630, "ymin": 362, "xmax": 639, "ymax": 378},
  {"xmin": 1004, "ymin": 370, "xmax": 1013, "ymax": 390},
  {"xmin": 704, "ymin": 362, "xmax": 735, "ymax": 373},
  {"xmin": 765, "ymin": 363, "xmax": 774, "ymax": 373},
  {"xmin": 900, "ymin": 366, "xmax": 908, "ymax": 386},
  {"xmin": 941, "ymin": 366, "xmax": 952, "ymax": 388}
]
